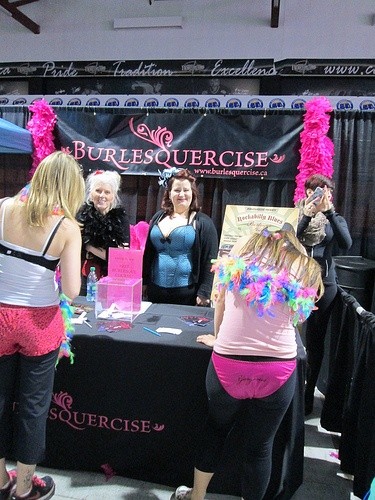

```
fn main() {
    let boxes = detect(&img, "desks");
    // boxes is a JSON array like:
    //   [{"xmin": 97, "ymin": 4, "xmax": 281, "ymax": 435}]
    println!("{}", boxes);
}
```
[{"xmin": 8, "ymin": 295, "xmax": 304, "ymax": 500}]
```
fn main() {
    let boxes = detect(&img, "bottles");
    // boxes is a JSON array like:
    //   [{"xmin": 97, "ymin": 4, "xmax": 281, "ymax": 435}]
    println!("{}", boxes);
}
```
[{"xmin": 87, "ymin": 267, "xmax": 98, "ymax": 301}]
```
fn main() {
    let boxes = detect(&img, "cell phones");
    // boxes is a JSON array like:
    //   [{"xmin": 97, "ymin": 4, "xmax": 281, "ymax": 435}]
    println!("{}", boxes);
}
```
[{"xmin": 311, "ymin": 187, "xmax": 324, "ymax": 205}]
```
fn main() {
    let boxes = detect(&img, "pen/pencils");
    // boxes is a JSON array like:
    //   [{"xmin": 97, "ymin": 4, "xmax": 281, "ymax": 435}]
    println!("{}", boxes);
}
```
[
  {"xmin": 84, "ymin": 321, "xmax": 92, "ymax": 329},
  {"xmin": 144, "ymin": 327, "xmax": 161, "ymax": 337}
]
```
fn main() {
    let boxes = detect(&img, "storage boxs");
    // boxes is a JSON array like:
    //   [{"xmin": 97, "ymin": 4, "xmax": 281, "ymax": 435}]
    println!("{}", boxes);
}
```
[{"xmin": 94, "ymin": 275, "xmax": 142, "ymax": 324}]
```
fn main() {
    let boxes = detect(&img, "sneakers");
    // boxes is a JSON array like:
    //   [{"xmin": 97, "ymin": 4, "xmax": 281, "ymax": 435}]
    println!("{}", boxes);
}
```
[
  {"xmin": 0, "ymin": 468, "xmax": 55, "ymax": 500},
  {"xmin": 175, "ymin": 485, "xmax": 193, "ymax": 500}
]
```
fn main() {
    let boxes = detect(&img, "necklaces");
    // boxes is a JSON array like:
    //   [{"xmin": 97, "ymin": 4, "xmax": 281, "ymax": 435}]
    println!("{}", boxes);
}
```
[{"xmin": 172, "ymin": 213, "xmax": 180, "ymax": 218}]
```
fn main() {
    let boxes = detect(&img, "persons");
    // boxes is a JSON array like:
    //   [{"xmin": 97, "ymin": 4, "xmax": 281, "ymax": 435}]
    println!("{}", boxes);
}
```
[
  {"xmin": 175, "ymin": 230, "xmax": 325, "ymax": 500},
  {"xmin": 297, "ymin": 174, "xmax": 353, "ymax": 415},
  {"xmin": 0, "ymin": 152, "xmax": 85, "ymax": 500},
  {"xmin": 142, "ymin": 168, "xmax": 219, "ymax": 307},
  {"xmin": 75, "ymin": 171, "xmax": 130, "ymax": 297}
]
